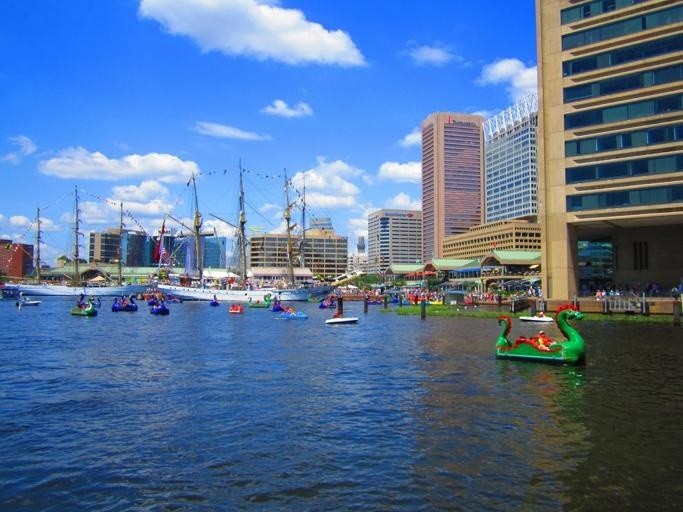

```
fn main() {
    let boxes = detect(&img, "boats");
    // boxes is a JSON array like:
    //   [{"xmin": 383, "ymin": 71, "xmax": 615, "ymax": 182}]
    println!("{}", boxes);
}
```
[
  {"xmin": 15, "ymin": 300, "xmax": 43, "ymax": 307},
  {"xmin": 71, "ymin": 297, "xmax": 101, "ymax": 317},
  {"xmin": 366, "ymin": 293, "xmax": 399, "ymax": 305},
  {"xmin": 497, "ymin": 301, "xmax": 586, "ymax": 368},
  {"xmin": 142, "ymin": 287, "xmax": 183, "ymax": 314},
  {"xmin": 113, "ymin": 295, "xmax": 138, "ymax": 312},
  {"xmin": 210, "ymin": 295, "xmax": 221, "ymax": 307},
  {"xmin": 308, "ymin": 294, "xmax": 358, "ymax": 325},
  {"xmin": 247, "ymin": 293, "xmax": 307, "ymax": 320},
  {"xmin": 229, "ymin": 304, "xmax": 245, "ymax": 314},
  {"xmin": 1, "ymin": 185, "xmax": 154, "ymax": 296}
]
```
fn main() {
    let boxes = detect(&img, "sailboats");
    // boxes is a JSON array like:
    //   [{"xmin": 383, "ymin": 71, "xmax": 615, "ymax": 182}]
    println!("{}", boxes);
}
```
[{"xmin": 154, "ymin": 156, "xmax": 339, "ymax": 306}]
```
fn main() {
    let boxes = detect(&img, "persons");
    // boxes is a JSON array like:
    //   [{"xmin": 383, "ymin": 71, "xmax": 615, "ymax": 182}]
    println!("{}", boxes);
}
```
[
  {"xmin": 536, "ymin": 331, "xmax": 556, "ymax": 349},
  {"xmin": 595, "ymin": 288, "xmax": 625, "ymax": 301},
  {"xmin": 286, "ymin": 306, "xmax": 293, "ymax": 313},
  {"xmin": 152, "ymin": 274, "xmax": 158, "ymax": 288},
  {"xmin": 275, "ymin": 291, "xmax": 282, "ymax": 305},
  {"xmin": 120, "ymin": 295, "xmax": 128, "ymax": 306}
]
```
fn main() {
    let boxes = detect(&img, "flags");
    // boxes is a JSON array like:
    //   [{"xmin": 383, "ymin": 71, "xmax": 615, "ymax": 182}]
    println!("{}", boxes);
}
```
[{"xmin": 153, "ymin": 224, "xmax": 166, "ymax": 263}]
